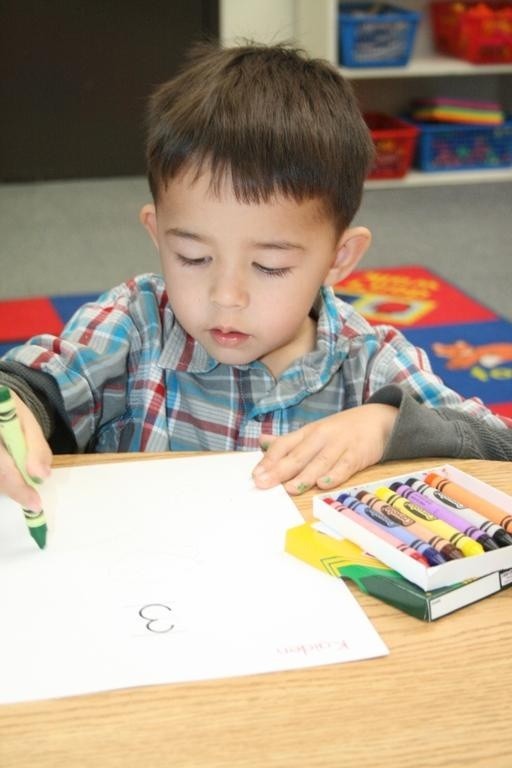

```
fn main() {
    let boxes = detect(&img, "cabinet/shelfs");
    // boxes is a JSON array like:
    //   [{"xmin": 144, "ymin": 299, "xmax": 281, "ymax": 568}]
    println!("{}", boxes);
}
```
[{"xmin": 291, "ymin": 0, "xmax": 510, "ymax": 184}]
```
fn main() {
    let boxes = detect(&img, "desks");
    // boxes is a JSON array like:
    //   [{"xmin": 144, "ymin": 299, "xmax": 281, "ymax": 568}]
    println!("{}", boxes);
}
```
[{"xmin": 0, "ymin": 447, "xmax": 509, "ymax": 765}]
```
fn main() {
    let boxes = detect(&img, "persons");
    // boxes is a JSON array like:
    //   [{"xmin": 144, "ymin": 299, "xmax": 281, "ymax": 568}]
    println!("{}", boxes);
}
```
[{"xmin": 1, "ymin": 41, "xmax": 512, "ymax": 511}]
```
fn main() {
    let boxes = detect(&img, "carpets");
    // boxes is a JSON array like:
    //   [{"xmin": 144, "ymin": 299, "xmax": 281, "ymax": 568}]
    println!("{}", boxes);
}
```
[{"xmin": 0, "ymin": 254, "xmax": 510, "ymax": 455}]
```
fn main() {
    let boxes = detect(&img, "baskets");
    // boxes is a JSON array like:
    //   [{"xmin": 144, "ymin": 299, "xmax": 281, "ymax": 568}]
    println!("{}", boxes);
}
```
[
  {"xmin": 359, "ymin": 111, "xmax": 418, "ymax": 178},
  {"xmin": 339, "ymin": 4, "xmax": 419, "ymax": 67},
  {"xmin": 399, "ymin": 112, "xmax": 512, "ymax": 172},
  {"xmin": 427, "ymin": 0, "xmax": 512, "ymax": 64}
]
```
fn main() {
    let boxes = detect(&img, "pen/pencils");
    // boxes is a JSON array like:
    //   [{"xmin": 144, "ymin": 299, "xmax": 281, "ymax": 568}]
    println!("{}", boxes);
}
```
[
  {"xmin": 322, "ymin": 471, "xmax": 512, "ymax": 570},
  {"xmin": 0, "ymin": 387, "xmax": 52, "ymax": 552}
]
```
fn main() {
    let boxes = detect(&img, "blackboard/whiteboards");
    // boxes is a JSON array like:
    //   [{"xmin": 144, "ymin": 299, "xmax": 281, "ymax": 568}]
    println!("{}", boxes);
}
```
[{"xmin": 1, "ymin": 2, "xmax": 220, "ymax": 187}]
